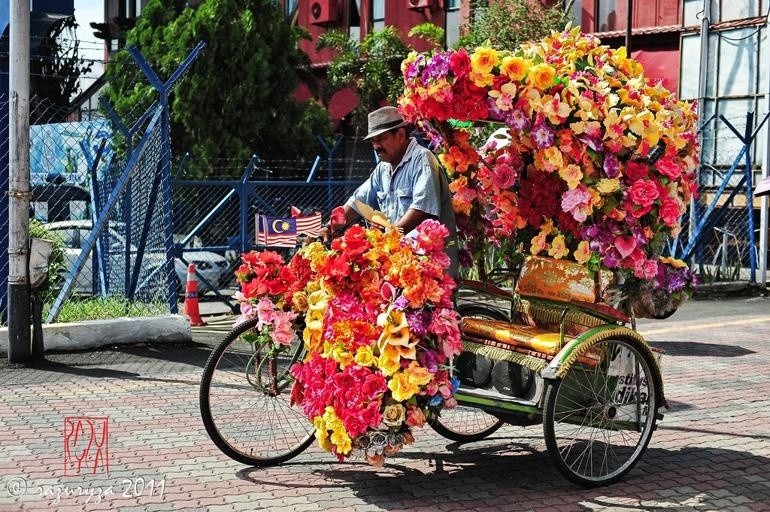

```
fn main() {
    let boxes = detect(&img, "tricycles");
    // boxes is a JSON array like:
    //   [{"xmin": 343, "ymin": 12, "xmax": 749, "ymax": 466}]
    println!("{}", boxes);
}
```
[{"xmin": 199, "ymin": 215, "xmax": 669, "ymax": 488}]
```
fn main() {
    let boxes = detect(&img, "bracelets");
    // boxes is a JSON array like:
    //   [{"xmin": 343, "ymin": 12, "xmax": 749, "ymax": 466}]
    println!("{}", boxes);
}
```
[{"xmin": 325, "ymin": 223, "xmax": 332, "ymax": 233}]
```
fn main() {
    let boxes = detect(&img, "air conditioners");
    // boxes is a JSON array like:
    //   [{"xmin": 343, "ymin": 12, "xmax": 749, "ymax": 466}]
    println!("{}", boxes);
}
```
[
  {"xmin": 308, "ymin": 0, "xmax": 339, "ymax": 25},
  {"xmin": 406, "ymin": 0, "xmax": 443, "ymax": 14}
]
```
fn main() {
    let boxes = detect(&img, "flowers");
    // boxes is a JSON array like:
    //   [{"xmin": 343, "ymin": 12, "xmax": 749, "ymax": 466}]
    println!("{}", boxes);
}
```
[{"xmin": 235, "ymin": 29, "xmax": 701, "ymax": 469}]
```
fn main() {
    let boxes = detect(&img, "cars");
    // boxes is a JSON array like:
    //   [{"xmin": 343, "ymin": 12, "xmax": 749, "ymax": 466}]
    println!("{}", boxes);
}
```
[{"xmin": 30, "ymin": 170, "xmax": 231, "ymax": 296}]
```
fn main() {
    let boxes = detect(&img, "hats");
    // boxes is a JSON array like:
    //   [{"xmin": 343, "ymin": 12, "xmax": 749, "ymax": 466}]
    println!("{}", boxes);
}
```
[{"xmin": 363, "ymin": 105, "xmax": 409, "ymax": 140}]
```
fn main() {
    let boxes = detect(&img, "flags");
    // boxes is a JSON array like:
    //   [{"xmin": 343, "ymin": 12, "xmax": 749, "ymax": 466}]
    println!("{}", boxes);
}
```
[{"xmin": 256, "ymin": 206, "xmax": 324, "ymax": 249}]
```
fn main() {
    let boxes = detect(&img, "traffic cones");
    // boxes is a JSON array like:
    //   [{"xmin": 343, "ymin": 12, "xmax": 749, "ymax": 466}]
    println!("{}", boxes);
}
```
[{"xmin": 182, "ymin": 264, "xmax": 208, "ymax": 327}]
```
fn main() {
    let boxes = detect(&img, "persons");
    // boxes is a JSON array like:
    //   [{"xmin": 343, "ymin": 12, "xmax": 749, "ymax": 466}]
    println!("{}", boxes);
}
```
[{"xmin": 304, "ymin": 106, "xmax": 460, "ymax": 435}]
